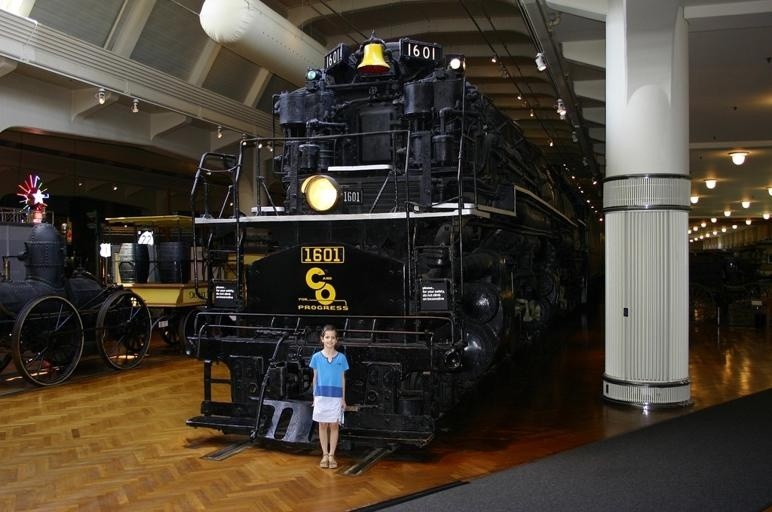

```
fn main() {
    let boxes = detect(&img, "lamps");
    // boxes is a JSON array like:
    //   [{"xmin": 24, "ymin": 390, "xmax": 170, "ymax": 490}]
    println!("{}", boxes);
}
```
[
  {"xmin": 98, "ymin": 88, "xmax": 106, "ymax": 105},
  {"xmin": 132, "ymin": 98, "xmax": 140, "ymax": 113},
  {"xmin": 217, "ymin": 125, "xmax": 224, "ymax": 138},
  {"xmin": 687, "ymin": 148, "xmax": 772, "ymax": 246},
  {"xmin": 239, "ymin": 132, "xmax": 248, "ymax": 148},
  {"xmin": 556, "ymin": 97, "xmax": 567, "ymax": 117},
  {"xmin": 535, "ymin": 52, "xmax": 546, "ymax": 72}
]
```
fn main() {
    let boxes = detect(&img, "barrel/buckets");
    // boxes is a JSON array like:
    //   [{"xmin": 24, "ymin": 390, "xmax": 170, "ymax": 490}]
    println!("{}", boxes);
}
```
[
  {"xmin": 119, "ymin": 243, "xmax": 149, "ymax": 283},
  {"xmin": 156, "ymin": 241, "xmax": 191, "ymax": 283}
]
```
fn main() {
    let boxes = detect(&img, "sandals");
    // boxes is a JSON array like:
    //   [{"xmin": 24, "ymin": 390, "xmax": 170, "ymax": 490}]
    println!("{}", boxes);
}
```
[
  {"xmin": 328, "ymin": 454, "xmax": 338, "ymax": 469},
  {"xmin": 319, "ymin": 453, "xmax": 329, "ymax": 469}
]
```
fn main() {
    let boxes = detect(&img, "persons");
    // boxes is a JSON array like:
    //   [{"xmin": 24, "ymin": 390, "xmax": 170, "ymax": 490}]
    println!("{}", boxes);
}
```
[{"xmin": 308, "ymin": 324, "xmax": 349, "ymax": 469}]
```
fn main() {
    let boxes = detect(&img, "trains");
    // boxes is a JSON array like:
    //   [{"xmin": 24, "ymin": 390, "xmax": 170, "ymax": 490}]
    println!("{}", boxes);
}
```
[
  {"xmin": 1, "ymin": 215, "xmax": 267, "ymax": 389},
  {"xmin": 182, "ymin": 27, "xmax": 604, "ymax": 452}
]
